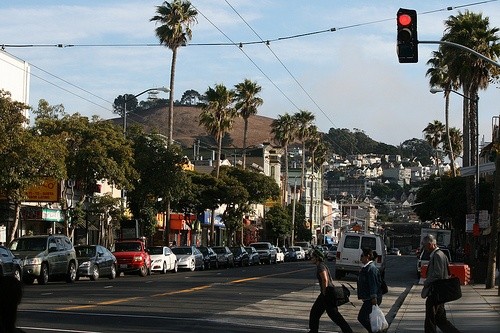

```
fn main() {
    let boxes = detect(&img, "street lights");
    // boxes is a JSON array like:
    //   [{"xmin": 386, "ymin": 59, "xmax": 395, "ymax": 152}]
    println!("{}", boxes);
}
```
[
  {"xmin": 430, "ymin": 87, "xmax": 480, "ymax": 283},
  {"xmin": 290, "ymin": 174, "xmax": 314, "ymax": 248},
  {"xmin": 121, "ymin": 86, "xmax": 171, "ymax": 217},
  {"xmin": 230, "ymin": 143, "xmax": 264, "ymax": 247}
]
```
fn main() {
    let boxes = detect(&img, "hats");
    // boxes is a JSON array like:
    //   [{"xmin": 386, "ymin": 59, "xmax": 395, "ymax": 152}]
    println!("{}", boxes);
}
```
[{"xmin": 313, "ymin": 249, "xmax": 324, "ymax": 262}]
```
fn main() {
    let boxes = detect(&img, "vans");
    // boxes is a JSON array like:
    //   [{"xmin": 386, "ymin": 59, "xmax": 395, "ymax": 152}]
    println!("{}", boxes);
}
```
[{"xmin": 334, "ymin": 233, "xmax": 385, "ymax": 280}]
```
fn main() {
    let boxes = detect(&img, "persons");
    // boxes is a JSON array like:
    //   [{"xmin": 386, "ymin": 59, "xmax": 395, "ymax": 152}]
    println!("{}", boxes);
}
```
[
  {"xmin": 308, "ymin": 249, "xmax": 353, "ymax": 333},
  {"xmin": 357, "ymin": 248, "xmax": 386, "ymax": 333},
  {"xmin": 477, "ymin": 245, "xmax": 483, "ymax": 263},
  {"xmin": 0, "ymin": 263, "xmax": 26, "ymax": 333},
  {"xmin": 421, "ymin": 235, "xmax": 460, "ymax": 333},
  {"xmin": 464, "ymin": 242, "xmax": 473, "ymax": 262},
  {"xmin": 484, "ymin": 243, "xmax": 490, "ymax": 258}
]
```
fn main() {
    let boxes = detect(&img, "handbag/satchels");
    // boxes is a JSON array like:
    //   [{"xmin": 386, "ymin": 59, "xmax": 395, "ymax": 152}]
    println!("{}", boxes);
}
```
[
  {"xmin": 334, "ymin": 284, "xmax": 350, "ymax": 306},
  {"xmin": 382, "ymin": 280, "xmax": 389, "ymax": 295},
  {"xmin": 431, "ymin": 277, "xmax": 462, "ymax": 306},
  {"xmin": 369, "ymin": 304, "xmax": 388, "ymax": 333}
]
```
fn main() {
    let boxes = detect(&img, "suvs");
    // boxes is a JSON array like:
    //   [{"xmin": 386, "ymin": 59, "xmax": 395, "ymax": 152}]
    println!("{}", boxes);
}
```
[
  {"xmin": 249, "ymin": 242, "xmax": 277, "ymax": 264},
  {"xmin": 111, "ymin": 238, "xmax": 151, "ymax": 277},
  {"xmin": 6, "ymin": 233, "xmax": 79, "ymax": 285},
  {"xmin": 295, "ymin": 242, "xmax": 312, "ymax": 261}
]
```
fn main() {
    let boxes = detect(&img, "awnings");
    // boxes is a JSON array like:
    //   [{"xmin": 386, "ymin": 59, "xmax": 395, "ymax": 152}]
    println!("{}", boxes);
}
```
[{"xmin": 76, "ymin": 219, "xmax": 98, "ymax": 230}]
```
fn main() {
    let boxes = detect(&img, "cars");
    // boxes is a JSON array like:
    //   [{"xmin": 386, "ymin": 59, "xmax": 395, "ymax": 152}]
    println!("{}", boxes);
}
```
[
  {"xmin": 72, "ymin": 245, "xmax": 119, "ymax": 280},
  {"xmin": 197, "ymin": 246, "xmax": 260, "ymax": 272},
  {"xmin": 273, "ymin": 246, "xmax": 305, "ymax": 263},
  {"xmin": 170, "ymin": 247, "xmax": 204, "ymax": 272},
  {"xmin": 410, "ymin": 244, "xmax": 452, "ymax": 275},
  {"xmin": 144, "ymin": 245, "xmax": 177, "ymax": 274},
  {"xmin": 389, "ymin": 248, "xmax": 400, "ymax": 255},
  {"xmin": 0, "ymin": 245, "xmax": 23, "ymax": 285}
]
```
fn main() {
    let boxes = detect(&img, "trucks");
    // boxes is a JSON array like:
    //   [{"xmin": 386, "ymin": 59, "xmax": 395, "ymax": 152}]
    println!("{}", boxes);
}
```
[{"xmin": 419, "ymin": 228, "xmax": 451, "ymax": 252}]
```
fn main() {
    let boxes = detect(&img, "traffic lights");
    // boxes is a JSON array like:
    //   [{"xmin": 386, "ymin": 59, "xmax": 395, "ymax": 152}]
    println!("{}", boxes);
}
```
[{"xmin": 396, "ymin": 7, "xmax": 419, "ymax": 63}]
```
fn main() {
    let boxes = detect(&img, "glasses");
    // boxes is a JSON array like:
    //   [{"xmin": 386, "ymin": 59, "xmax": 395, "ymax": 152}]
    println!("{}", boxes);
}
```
[{"xmin": 361, "ymin": 255, "xmax": 363, "ymax": 257}]
